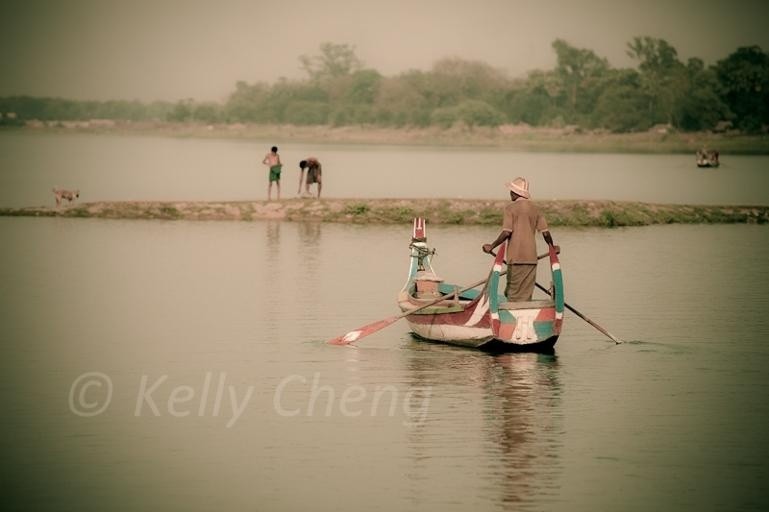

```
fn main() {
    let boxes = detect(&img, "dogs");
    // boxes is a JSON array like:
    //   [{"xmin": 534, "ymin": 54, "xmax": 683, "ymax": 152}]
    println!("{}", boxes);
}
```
[{"xmin": 51, "ymin": 186, "xmax": 80, "ymax": 210}]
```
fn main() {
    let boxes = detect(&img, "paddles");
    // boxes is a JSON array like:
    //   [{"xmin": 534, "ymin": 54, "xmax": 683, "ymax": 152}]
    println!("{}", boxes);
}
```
[
  {"xmin": 325, "ymin": 246, "xmax": 558, "ymax": 346},
  {"xmin": 481, "ymin": 245, "xmax": 622, "ymax": 344}
]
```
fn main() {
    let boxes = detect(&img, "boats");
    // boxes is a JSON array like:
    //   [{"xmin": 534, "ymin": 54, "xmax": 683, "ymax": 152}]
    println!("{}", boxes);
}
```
[
  {"xmin": 402, "ymin": 332, "xmax": 559, "ymax": 366},
  {"xmin": 697, "ymin": 158, "xmax": 720, "ymax": 167},
  {"xmin": 396, "ymin": 215, "xmax": 565, "ymax": 356}
]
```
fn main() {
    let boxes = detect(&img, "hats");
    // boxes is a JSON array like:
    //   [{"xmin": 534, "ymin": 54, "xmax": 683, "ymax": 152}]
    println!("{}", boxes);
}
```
[{"xmin": 506, "ymin": 176, "xmax": 531, "ymax": 199}]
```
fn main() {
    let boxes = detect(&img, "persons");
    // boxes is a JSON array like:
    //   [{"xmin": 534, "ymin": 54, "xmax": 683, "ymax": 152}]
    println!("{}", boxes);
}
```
[
  {"xmin": 297, "ymin": 154, "xmax": 323, "ymax": 200},
  {"xmin": 298, "ymin": 222, "xmax": 323, "ymax": 250},
  {"xmin": 711, "ymin": 149, "xmax": 720, "ymax": 162},
  {"xmin": 266, "ymin": 222, "xmax": 283, "ymax": 267},
  {"xmin": 489, "ymin": 358, "xmax": 555, "ymax": 511},
  {"xmin": 262, "ymin": 145, "xmax": 284, "ymax": 200},
  {"xmin": 699, "ymin": 149, "xmax": 709, "ymax": 159},
  {"xmin": 481, "ymin": 177, "xmax": 561, "ymax": 304}
]
